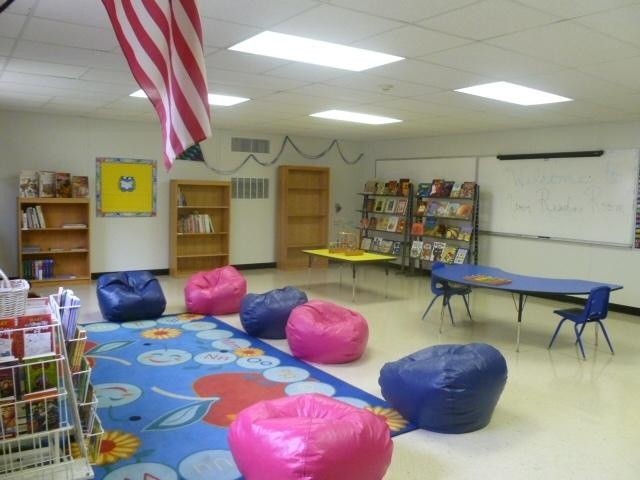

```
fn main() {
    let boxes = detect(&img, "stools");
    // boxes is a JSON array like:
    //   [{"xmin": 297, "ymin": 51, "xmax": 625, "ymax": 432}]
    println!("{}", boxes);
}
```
[
  {"xmin": 227, "ymin": 393, "xmax": 394, "ymax": 480},
  {"xmin": 379, "ymin": 343, "xmax": 508, "ymax": 434},
  {"xmin": 96, "ymin": 265, "xmax": 369, "ymax": 364}
]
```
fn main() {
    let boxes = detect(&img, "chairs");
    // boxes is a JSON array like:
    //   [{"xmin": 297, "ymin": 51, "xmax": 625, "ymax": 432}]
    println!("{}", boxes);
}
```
[
  {"xmin": 422, "ymin": 263, "xmax": 474, "ymax": 332},
  {"xmin": 548, "ymin": 286, "xmax": 614, "ymax": 360}
]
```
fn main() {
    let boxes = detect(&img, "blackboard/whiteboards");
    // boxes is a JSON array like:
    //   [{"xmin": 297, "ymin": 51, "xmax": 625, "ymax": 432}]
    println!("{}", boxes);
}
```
[{"xmin": 375, "ymin": 147, "xmax": 639, "ymax": 247}]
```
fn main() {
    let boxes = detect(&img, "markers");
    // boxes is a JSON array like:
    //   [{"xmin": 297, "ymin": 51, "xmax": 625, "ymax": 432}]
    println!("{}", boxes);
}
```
[{"xmin": 538, "ymin": 236, "xmax": 549, "ymax": 238}]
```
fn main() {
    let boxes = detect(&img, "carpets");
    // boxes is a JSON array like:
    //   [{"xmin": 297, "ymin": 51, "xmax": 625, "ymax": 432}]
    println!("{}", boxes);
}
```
[{"xmin": 78, "ymin": 312, "xmax": 419, "ymax": 480}]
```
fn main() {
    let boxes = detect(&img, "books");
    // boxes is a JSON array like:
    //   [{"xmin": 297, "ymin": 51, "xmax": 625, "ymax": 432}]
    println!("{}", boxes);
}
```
[
  {"xmin": 177, "ymin": 185, "xmax": 187, "ymax": 206},
  {"xmin": 0, "ymin": 287, "xmax": 102, "ymax": 474},
  {"xmin": 62, "ymin": 223, "xmax": 87, "ymax": 229},
  {"xmin": 23, "ymin": 259, "xmax": 54, "ymax": 280},
  {"xmin": 70, "ymin": 248, "xmax": 88, "ymax": 252},
  {"xmin": 48, "ymin": 248, "xmax": 63, "ymax": 253},
  {"xmin": 23, "ymin": 246, "xmax": 40, "ymax": 252},
  {"xmin": 178, "ymin": 211, "xmax": 214, "ymax": 233},
  {"xmin": 21, "ymin": 206, "xmax": 47, "ymax": 229},
  {"xmin": 360, "ymin": 179, "xmax": 475, "ymax": 264}
]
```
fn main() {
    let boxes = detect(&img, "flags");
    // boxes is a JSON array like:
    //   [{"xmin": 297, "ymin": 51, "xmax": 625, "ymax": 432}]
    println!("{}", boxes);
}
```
[{"xmin": 102, "ymin": 0, "xmax": 213, "ymax": 173}]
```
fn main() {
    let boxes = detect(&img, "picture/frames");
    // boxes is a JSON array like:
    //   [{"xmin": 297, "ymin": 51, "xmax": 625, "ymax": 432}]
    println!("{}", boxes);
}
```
[{"xmin": 95, "ymin": 157, "xmax": 158, "ymax": 217}]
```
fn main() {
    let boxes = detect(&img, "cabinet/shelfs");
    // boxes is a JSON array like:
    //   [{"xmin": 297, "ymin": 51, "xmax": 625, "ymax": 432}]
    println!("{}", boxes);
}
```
[
  {"xmin": 170, "ymin": 179, "xmax": 230, "ymax": 278},
  {"xmin": 17, "ymin": 197, "xmax": 92, "ymax": 288},
  {"xmin": 0, "ymin": 294, "xmax": 104, "ymax": 480},
  {"xmin": 276, "ymin": 165, "xmax": 480, "ymax": 272}
]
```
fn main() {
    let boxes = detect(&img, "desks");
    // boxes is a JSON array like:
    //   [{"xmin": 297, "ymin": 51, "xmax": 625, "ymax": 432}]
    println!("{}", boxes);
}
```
[
  {"xmin": 300, "ymin": 248, "xmax": 398, "ymax": 303},
  {"xmin": 432, "ymin": 264, "xmax": 623, "ymax": 352}
]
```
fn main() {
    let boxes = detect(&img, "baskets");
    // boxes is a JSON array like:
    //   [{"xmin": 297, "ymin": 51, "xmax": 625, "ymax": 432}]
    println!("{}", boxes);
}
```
[{"xmin": 0, "ymin": 269, "xmax": 31, "ymax": 320}]
